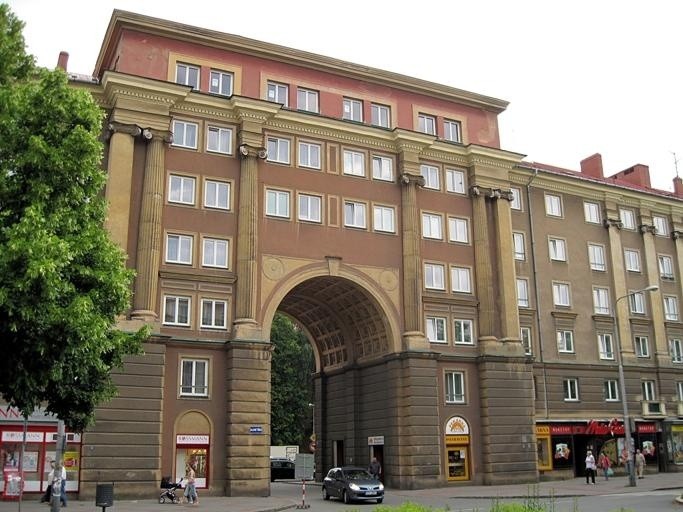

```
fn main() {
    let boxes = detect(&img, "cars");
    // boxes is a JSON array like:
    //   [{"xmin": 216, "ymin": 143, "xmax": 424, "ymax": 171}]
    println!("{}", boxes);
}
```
[
  {"xmin": 271, "ymin": 458, "xmax": 295, "ymax": 481},
  {"xmin": 322, "ymin": 467, "xmax": 385, "ymax": 504}
]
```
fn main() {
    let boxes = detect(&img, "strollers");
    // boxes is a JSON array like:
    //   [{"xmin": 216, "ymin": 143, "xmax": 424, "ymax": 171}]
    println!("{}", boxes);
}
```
[{"xmin": 159, "ymin": 476, "xmax": 185, "ymax": 503}]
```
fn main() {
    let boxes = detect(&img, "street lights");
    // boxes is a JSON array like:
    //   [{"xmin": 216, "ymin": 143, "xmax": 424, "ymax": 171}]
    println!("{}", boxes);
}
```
[
  {"xmin": 613, "ymin": 286, "xmax": 658, "ymax": 487},
  {"xmin": 309, "ymin": 403, "xmax": 316, "ymax": 434}
]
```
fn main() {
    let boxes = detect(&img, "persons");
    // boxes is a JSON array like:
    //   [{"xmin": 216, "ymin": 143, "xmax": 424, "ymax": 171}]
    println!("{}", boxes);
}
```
[
  {"xmin": 368, "ymin": 458, "xmax": 381, "ymax": 480},
  {"xmin": 585, "ymin": 450, "xmax": 595, "ymax": 485},
  {"xmin": 167, "ymin": 462, "xmax": 200, "ymax": 505},
  {"xmin": 622, "ymin": 446, "xmax": 631, "ymax": 474},
  {"xmin": 60, "ymin": 466, "xmax": 68, "ymax": 507},
  {"xmin": 635, "ymin": 449, "xmax": 646, "ymax": 479},
  {"xmin": 599, "ymin": 452, "xmax": 611, "ymax": 481},
  {"xmin": 44, "ymin": 461, "xmax": 55, "ymax": 503}
]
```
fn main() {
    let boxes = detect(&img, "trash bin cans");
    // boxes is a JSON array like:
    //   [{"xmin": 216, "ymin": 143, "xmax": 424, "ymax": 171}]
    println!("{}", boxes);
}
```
[{"xmin": 96, "ymin": 484, "xmax": 113, "ymax": 512}]
